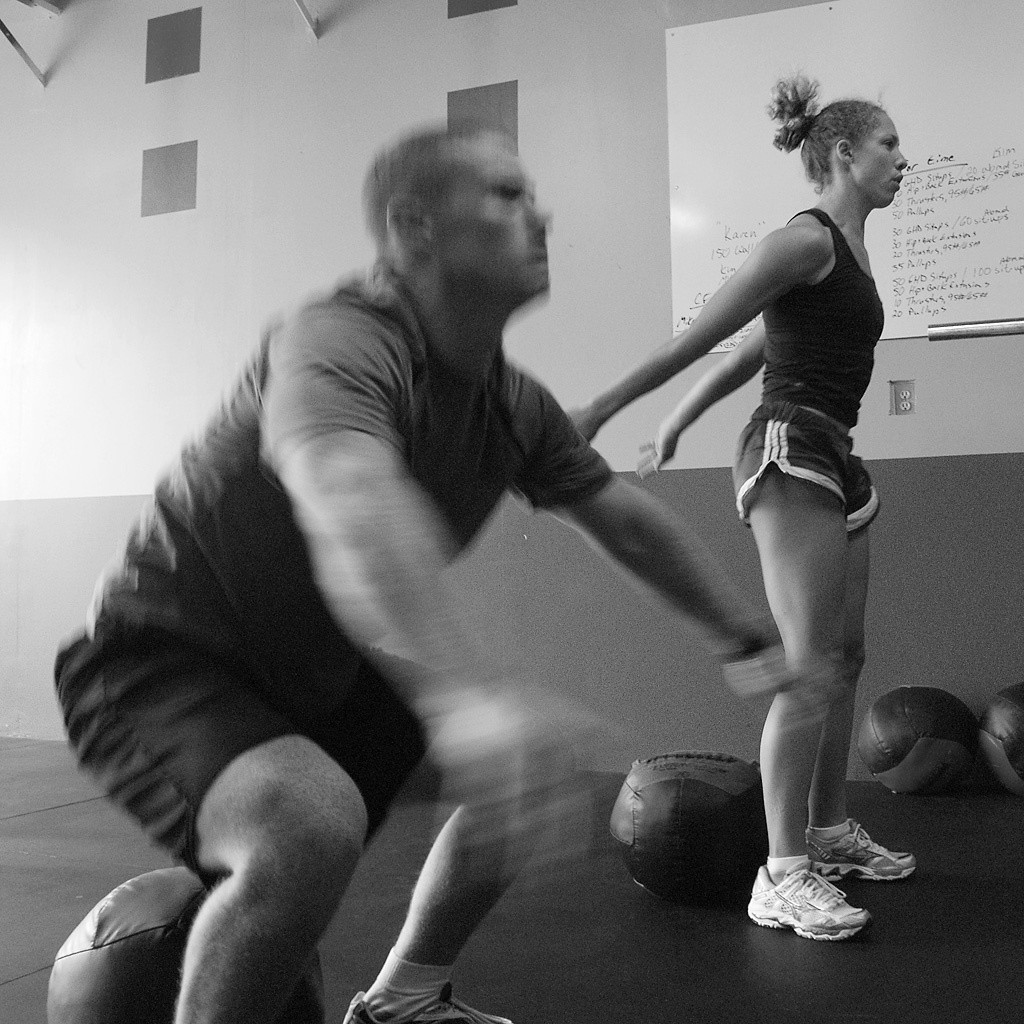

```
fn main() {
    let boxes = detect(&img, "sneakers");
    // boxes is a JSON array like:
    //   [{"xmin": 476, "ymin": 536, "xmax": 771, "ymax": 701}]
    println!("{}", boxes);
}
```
[
  {"xmin": 747, "ymin": 865, "xmax": 870, "ymax": 941},
  {"xmin": 805, "ymin": 820, "xmax": 916, "ymax": 881},
  {"xmin": 342, "ymin": 981, "xmax": 513, "ymax": 1024}
]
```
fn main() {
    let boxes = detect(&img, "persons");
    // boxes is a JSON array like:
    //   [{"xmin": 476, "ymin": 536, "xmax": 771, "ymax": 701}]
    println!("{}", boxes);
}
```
[
  {"xmin": 55, "ymin": 117, "xmax": 840, "ymax": 1024},
  {"xmin": 569, "ymin": 75, "xmax": 917, "ymax": 942}
]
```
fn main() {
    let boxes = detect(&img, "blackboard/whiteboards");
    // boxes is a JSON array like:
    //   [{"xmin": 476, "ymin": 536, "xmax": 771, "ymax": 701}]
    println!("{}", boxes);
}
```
[{"xmin": 664, "ymin": 0, "xmax": 1024, "ymax": 355}]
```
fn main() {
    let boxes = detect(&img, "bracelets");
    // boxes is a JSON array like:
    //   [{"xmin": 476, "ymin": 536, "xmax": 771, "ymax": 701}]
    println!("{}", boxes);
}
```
[{"xmin": 713, "ymin": 636, "xmax": 770, "ymax": 667}]
